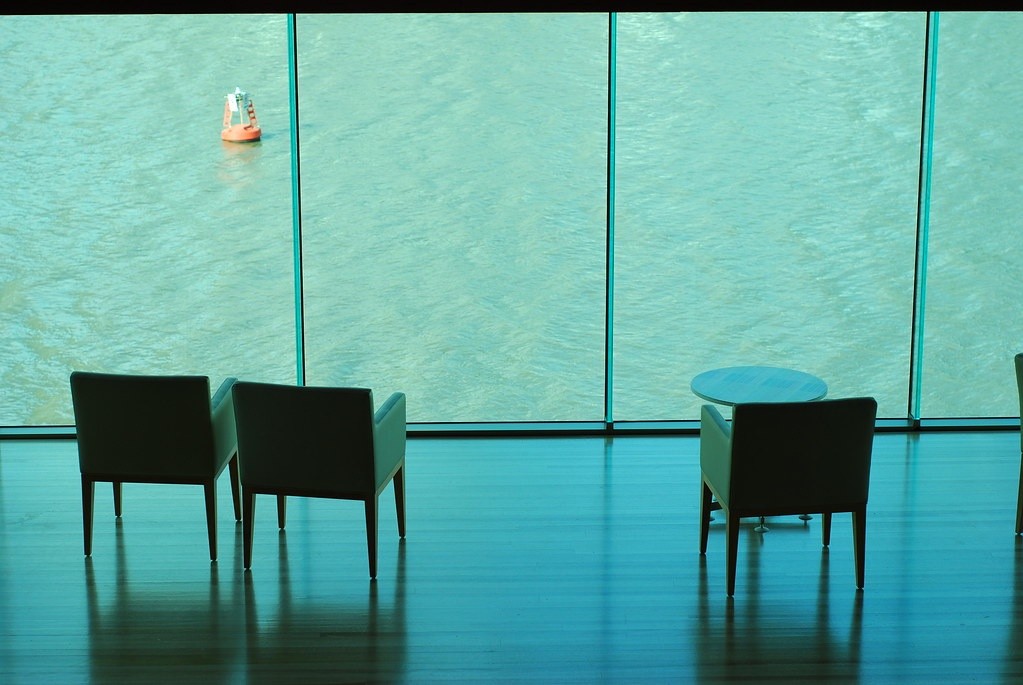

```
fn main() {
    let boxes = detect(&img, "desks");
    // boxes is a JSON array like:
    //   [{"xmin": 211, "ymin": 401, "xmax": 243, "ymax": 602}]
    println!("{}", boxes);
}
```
[{"xmin": 691, "ymin": 366, "xmax": 828, "ymax": 532}]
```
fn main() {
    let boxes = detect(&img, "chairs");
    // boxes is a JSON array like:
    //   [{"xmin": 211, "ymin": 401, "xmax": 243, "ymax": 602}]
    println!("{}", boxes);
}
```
[
  {"xmin": 70, "ymin": 370, "xmax": 241, "ymax": 561},
  {"xmin": 698, "ymin": 397, "xmax": 877, "ymax": 597},
  {"xmin": 232, "ymin": 381, "xmax": 407, "ymax": 580}
]
